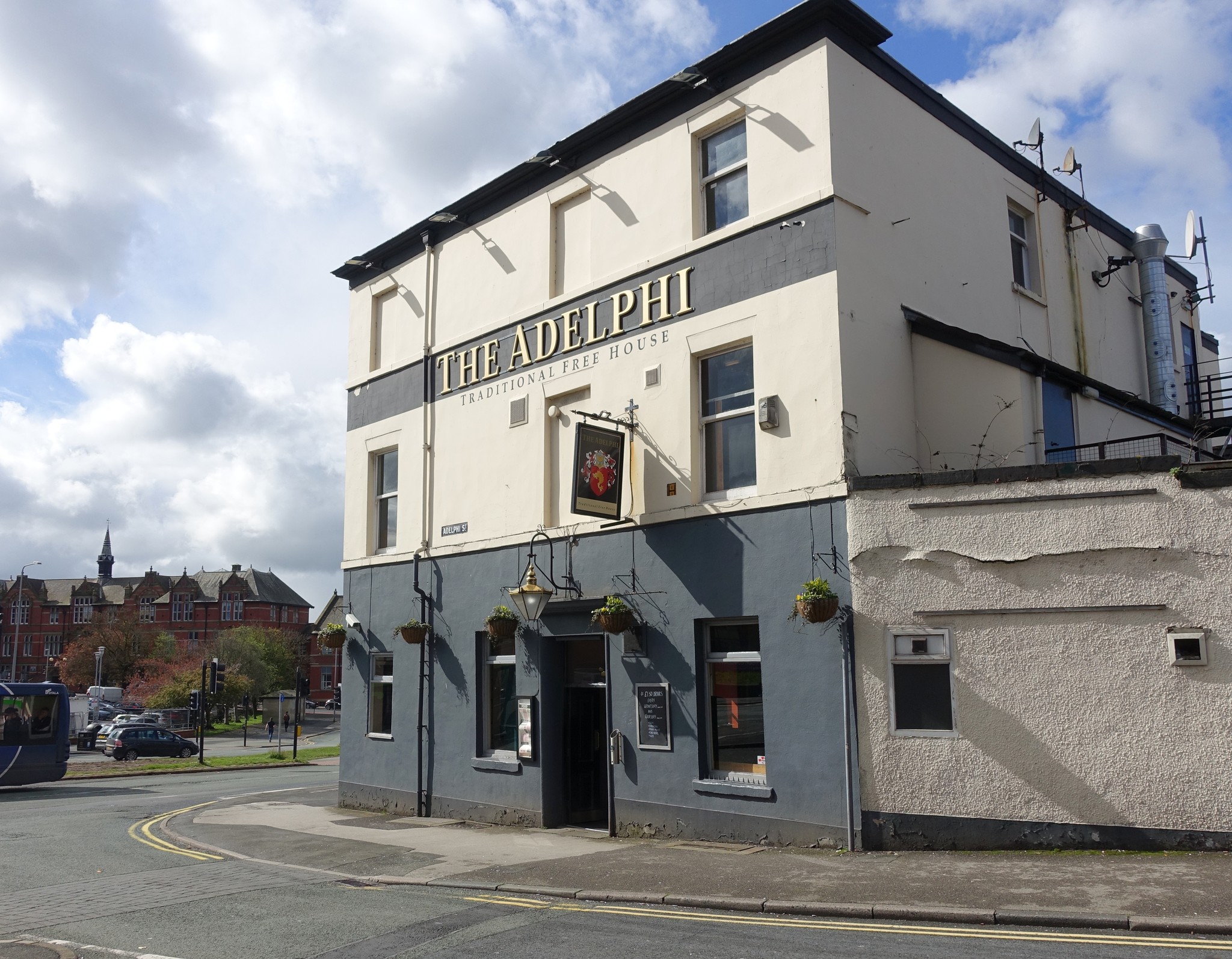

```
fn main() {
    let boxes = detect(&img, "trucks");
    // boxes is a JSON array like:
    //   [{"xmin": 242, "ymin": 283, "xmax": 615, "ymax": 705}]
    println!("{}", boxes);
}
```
[
  {"xmin": 90, "ymin": 686, "xmax": 127, "ymax": 703},
  {"xmin": 212, "ymin": 702, "xmax": 225, "ymax": 721}
]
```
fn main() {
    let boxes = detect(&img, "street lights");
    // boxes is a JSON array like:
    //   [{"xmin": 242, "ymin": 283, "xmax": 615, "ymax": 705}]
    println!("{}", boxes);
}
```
[
  {"xmin": 10, "ymin": 561, "xmax": 42, "ymax": 684},
  {"xmin": 96, "ymin": 645, "xmax": 106, "ymax": 720},
  {"xmin": 94, "ymin": 651, "xmax": 100, "ymax": 699}
]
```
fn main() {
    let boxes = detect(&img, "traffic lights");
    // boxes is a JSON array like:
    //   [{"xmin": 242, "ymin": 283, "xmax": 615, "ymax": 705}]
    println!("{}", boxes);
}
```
[
  {"xmin": 241, "ymin": 688, "xmax": 252, "ymax": 747},
  {"xmin": 333, "ymin": 682, "xmax": 341, "ymax": 706},
  {"xmin": 209, "ymin": 658, "xmax": 226, "ymax": 695},
  {"xmin": 189, "ymin": 692, "xmax": 196, "ymax": 710}
]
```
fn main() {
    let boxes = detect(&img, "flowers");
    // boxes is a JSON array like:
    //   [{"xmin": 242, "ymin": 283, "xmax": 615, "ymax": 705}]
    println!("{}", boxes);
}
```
[
  {"xmin": 585, "ymin": 594, "xmax": 632, "ymax": 634},
  {"xmin": 785, "ymin": 574, "xmax": 840, "ymax": 634},
  {"xmin": 317, "ymin": 622, "xmax": 346, "ymax": 650}
]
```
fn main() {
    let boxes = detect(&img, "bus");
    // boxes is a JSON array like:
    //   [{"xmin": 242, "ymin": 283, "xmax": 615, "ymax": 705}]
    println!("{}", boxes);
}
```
[{"xmin": 1, "ymin": 680, "xmax": 71, "ymax": 787}]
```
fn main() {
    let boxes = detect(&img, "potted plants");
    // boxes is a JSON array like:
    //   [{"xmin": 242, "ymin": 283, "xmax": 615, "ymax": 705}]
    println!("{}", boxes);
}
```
[
  {"xmin": 391, "ymin": 618, "xmax": 433, "ymax": 644},
  {"xmin": 482, "ymin": 605, "xmax": 523, "ymax": 648}
]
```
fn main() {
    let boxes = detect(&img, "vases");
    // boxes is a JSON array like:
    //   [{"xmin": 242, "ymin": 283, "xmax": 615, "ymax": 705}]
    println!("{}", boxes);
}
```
[
  {"xmin": 321, "ymin": 631, "xmax": 345, "ymax": 648},
  {"xmin": 598, "ymin": 612, "xmax": 628, "ymax": 633},
  {"xmin": 796, "ymin": 599, "xmax": 839, "ymax": 623}
]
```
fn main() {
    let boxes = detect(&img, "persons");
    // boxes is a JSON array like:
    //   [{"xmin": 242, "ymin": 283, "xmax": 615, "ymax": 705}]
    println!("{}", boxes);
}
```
[
  {"xmin": 283, "ymin": 711, "xmax": 290, "ymax": 732},
  {"xmin": 3, "ymin": 707, "xmax": 22, "ymax": 740},
  {"xmin": 34, "ymin": 707, "xmax": 51, "ymax": 733},
  {"xmin": 265, "ymin": 717, "xmax": 275, "ymax": 742}
]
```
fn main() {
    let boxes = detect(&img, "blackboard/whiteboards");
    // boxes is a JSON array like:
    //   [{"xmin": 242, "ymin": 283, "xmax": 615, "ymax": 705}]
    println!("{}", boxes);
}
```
[{"xmin": 630, "ymin": 682, "xmax": 672, "ymax": 751}]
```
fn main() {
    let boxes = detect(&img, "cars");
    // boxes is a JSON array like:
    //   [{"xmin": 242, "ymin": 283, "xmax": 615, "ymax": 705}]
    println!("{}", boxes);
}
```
[
  {"xmin": 95, "ymin": 723, "xmax": 158, "ymax": 758},
  {"xmin": 86, "ymin": 686, "xmax": 102, "ymax": 697},
  {"xmin": 97, "ymin": 703, "xmax": 127, "ymax": 717},
  {"xmin": 154, "ymin": 710, "xmax": 181, "ymax": 722},
  {"xmin": 68, "ymin": 690, "xmax": 76, "ymax": 697},
  {"xmin": 89, "ymin": 706, "xmax": 111, "ymax": 721},
  {"xmin": 141, "ymin": 713, "xmax": 171, "ymax": 725},
  {"xmin": 111, "ymin": 701, "xmax": 145, "ymax": 715},
  {"xmin": 305, "ymin": 700, "xmax": 319, "ymax": 709},
  {"xmin": 199, "ymin": 708, "xmax": 214, "ymax": 723},
  {"xmin": 103, "ymin": 727, "xmax": 200, "ymax": 760},
  {"xmin": 112, "ymin": 713, "xmax": 143, "ymax": 723},
  {"xmin": 324, "ymin": 698, "xmax": 341, "ymax": 710},
  {"xmin": 119, "ymin": 717, "xmax": 160, "ymax": 728}
]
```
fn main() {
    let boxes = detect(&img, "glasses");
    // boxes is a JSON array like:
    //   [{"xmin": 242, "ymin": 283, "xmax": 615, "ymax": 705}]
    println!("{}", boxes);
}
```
[{"xmin": 40, "ymin": 711, "xmax": 46, "ymax": 713}]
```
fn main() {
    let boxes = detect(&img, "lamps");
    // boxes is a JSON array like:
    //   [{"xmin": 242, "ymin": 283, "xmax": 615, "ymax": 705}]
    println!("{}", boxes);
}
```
[
  {"xmin": 500, "ymin": 523, "xmax": 582, "ymax": 624},
  {"xmin": 669, "ymin": 66, "xmax": 723, "ymax": 99},
  {"xmin": 526, "ymin": 150, "xmax": 577, "ymax": 174},
  {"xmin": 344, "ymin": 256, "xmax": 388, "ymax": 271},
  {"xmin": 429, "ymin": 211, "xmax": 472, "ymax": 228}
]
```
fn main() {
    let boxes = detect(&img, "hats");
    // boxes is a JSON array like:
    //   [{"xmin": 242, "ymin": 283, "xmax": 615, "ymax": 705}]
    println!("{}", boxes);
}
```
[
  {"xmin": 5, "ymin": 707, "xmax": 18, "ymax": 714},
  {"xmin": 40, "ymin": 707, "xmax": 49, "ymax": 710}
]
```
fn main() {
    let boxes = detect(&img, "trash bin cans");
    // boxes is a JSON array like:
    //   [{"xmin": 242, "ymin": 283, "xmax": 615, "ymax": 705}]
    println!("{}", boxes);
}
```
[
  {"xmin": 76, "ymin": 730, "xmax": 93, "ymax": 751},
  {"xmin": 296, "ymin": 726, "xmax": 301, "ymax": 736}
]
